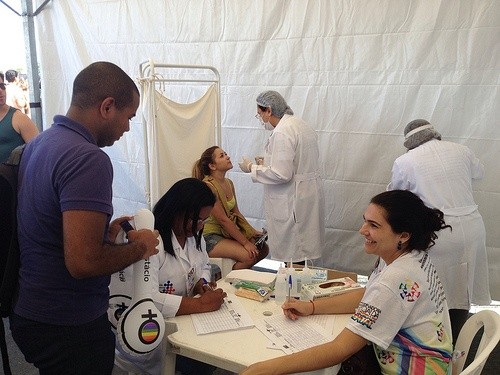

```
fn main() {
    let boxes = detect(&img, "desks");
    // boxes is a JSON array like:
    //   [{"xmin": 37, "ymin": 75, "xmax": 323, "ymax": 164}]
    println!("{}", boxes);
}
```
[{"xmin": 160, "ymin": 276, "xmax": 356, "ymax": 375}]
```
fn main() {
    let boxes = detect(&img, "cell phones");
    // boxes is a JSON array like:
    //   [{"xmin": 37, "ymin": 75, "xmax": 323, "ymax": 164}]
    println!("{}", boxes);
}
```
[{"xmin": 255, "ymin": 233, "xmax": 268, "ymax": 246}]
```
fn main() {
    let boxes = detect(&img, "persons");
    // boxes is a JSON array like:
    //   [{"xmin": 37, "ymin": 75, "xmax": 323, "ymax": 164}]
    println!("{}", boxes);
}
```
[
  {"xmin": 9, "ymin": 61, "xmax": 159, "ymax": 375},
  {"xmin": 387, "ymin": 118, "xmax": 491, "ymax": 347},
  {"xmin": 238, "ymin": 90, "xmax": 325, "ymax": 265},
  {"xmin": 238, "ymin": 189, "xmax": 453, "ymax": 375},
  {"xmin": 0, "ymin": 69, "xmax": 40, "ymax": 161},
  {"xmin": 106, "ymin": 177, "xmax": 227, "ymax": 375},
  {"xmin": 192, "ymin": 146, "xmax": 270, "ymax": 270}
]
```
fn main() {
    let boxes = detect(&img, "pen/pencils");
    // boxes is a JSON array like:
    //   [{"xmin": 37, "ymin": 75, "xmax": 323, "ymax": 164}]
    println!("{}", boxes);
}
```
[
  {"xmin": 289, "ymin": 275, "xmax": 292, "ymax": 301},
  {"xmin": 270, "ymin": 295, "xmax": 299, "ymax": 300},
  {"xmin": 203, "ymin": 278, "xmax": 214, "ymax": 292}
]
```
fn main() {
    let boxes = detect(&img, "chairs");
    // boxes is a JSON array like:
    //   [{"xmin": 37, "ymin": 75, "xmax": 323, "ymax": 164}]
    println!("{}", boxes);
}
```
[{"xmin": 452, "ymin": 310, "xmax": 500, "ymax": 375}]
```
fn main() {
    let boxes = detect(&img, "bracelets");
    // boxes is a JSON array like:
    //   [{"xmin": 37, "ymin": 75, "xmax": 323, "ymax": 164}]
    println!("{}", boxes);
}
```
[{"xmin": 310, "ymin": 300, "xmax": 315, "ymax": 315}]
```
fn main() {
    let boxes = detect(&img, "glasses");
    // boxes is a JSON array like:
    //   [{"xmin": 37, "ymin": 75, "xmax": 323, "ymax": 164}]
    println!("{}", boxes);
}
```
[
  {"xmin": 255, "ymin": 111, "xmax": 262, "ymax": 119},
  {"xmin": 0, "ymin": 83, "xmax": 7, "ymax": 90},
  {"xmin": 190, "ymin": 215, "xmax": 211, "ymax": 225}
]
